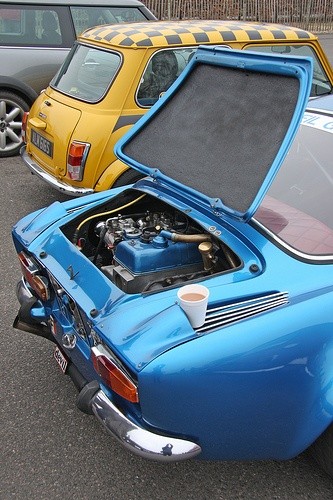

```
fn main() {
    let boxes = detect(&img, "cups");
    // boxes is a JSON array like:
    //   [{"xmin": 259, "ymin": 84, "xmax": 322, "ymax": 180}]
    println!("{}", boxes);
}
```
[{"xmin": 177, "ymin": 283, "xmax": 209, "ymax": 328}]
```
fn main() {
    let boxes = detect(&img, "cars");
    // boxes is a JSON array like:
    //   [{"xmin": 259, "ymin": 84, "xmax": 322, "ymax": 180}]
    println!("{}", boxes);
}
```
[
  {"xmin": 9, "ymin": 45, "xmax": 333, "ymax": 472},
  {"xmin": 19, "ymin": 19, "xmax": 333, "ymax": 198}
]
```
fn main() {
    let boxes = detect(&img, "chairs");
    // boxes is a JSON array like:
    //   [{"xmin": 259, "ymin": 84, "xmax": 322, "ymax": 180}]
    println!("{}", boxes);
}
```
[
  {"xmin": 140, "ymin": 50, "xmax": 178, "ymax": 98},
  {"xmin": 41, "ymin": 12, "xmax": 61, "ymax": 43}
]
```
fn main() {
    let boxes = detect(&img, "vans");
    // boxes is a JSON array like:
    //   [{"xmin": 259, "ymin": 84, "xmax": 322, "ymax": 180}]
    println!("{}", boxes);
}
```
[{"xmin": 0, "ymin": 1, "xmax": 159, "ymax": 159}]
